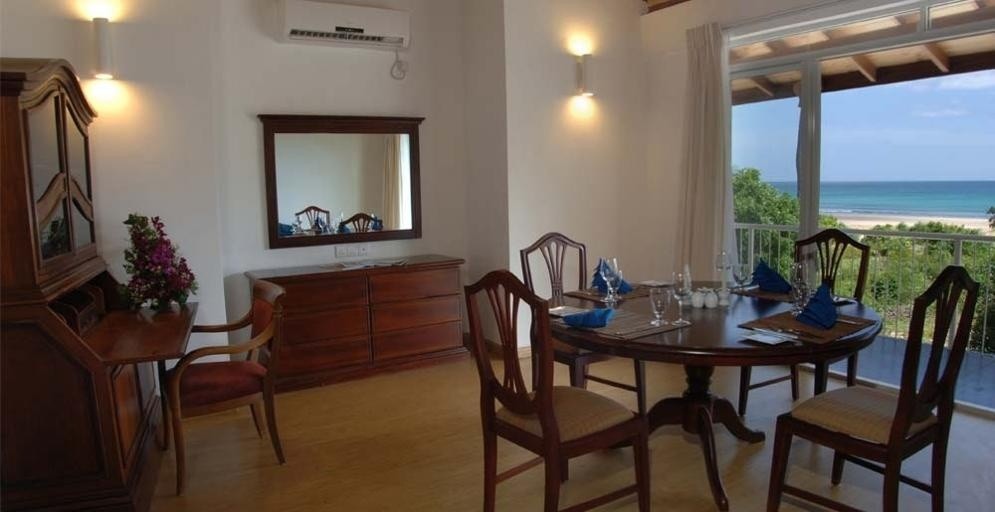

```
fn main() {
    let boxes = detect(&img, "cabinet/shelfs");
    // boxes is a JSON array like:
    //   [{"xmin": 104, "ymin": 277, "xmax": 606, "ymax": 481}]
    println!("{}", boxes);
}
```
[
  {"xmin": 1, "ymin": 52, "xmax": 132, "ymax": 298},
  {"xmin": 0, "ymin": 268, "xmax": 201, "ymax": 512},
  {"xmin": 241, "ymin": 251, "xmax": 473, "ymax": 399}
]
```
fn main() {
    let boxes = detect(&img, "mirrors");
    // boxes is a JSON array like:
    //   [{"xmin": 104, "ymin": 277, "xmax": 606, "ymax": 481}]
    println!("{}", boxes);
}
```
[{"xmin": 254, "ymin": 110, "xmax": 425, "ymax": 250}]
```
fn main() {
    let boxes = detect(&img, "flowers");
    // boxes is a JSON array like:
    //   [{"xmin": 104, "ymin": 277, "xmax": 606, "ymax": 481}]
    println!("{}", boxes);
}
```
[{"xmin": 112, "ymin": 209, "xmax": 198, "ymax": 314}]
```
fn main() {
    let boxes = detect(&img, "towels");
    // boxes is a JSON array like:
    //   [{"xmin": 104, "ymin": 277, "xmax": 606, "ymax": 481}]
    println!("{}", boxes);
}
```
[
  {"xmin": 794, "ymin": 281, "xmax": 842, "ymax": 333},
  {"xmin": 587, "ymin": 255, "xmax": 634, "ymax": 296},
  {"xmin": 336, "ymin": 221, "xmax": 350, "ymax": 234},
  {"xmin": 277, "ymin": 221, "xmax": 294, "ymax": 234},
  {"xmin": 371, "ymin": 214, "xmax": 384, "ymax": 231},
  {"xmin": 560, "ymin": 298, "xmax": 616, "ymax": 329},
  {"xmin": 312, "ymin": 216, "xmax": 328, "ymax": 233},
  {"xmin": 748, "ymin": 253, "xmax": 795, "ymax": 298}
]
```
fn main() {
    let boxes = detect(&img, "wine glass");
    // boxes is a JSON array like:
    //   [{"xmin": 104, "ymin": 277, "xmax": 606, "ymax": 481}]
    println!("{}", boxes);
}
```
[
  {"xmin": 290, "ymin": 210, "xmax": 379, "ymax": 236},
  {"xmin": 598, "ymin": 249, "xmax": 808, "ymax": 328}
]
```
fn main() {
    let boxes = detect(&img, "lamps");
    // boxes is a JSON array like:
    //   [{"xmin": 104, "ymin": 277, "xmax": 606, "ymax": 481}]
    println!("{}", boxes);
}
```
[
  {"xmin": 576, "ymin": 52, "xmax": 597, "ymax": 100},
  {"xmin": 91, "ymin": 13, "xmax": 115, "ymax": 84}
]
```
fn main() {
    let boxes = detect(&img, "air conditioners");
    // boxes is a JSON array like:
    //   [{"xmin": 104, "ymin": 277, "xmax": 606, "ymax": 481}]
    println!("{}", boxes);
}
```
[{"xmin": 280, "ymin": 2, "xmax": 412, "ymax": 56}]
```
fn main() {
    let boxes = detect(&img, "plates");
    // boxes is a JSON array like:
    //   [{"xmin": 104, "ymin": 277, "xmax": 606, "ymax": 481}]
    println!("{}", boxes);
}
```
[
  {"xmin": 743, "ymin": 331, "xmax": 801, "ymax": 345},
  {"xmin": 546, "ymin": 305, "xmax": 592, "ymax": 319}
]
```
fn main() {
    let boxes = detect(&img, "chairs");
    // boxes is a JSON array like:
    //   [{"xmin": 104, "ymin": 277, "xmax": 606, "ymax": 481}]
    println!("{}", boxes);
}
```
[
  {"xmin": 459, "ymin": 265, "xmax": 655, "ymax": 512},
  {"xmin": 338, "ymin": 210, "xmax": 383, "ymax": 233},
  {"xmin": 764, "ymin": 262, "xmax": 982, "ymax": 510},
  {"xmin": 517, "ymin": 229, "xmax": 652, "ymax": 416},
  {"xmin": 736, "ymin": 227, "xmax": 873, "ymax": 420},
  {"xmin": 151, "ymin": 277, "xmax": 292, "ymax": 498},
  {"xmin": 293, "ymin": 205, "xmax": 333, "ymax": 230}
]
